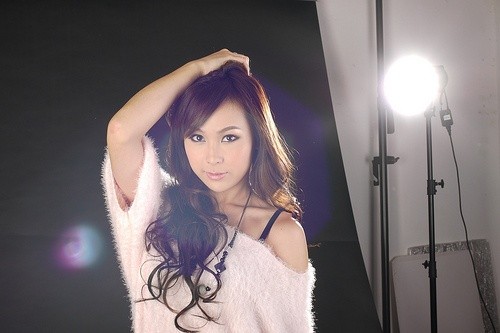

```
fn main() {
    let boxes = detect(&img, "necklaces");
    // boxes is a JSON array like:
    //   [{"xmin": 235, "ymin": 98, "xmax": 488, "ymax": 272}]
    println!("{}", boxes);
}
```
[{"xmin": 205, "ymin": 186, "xmax": 252, "ymax": 277}]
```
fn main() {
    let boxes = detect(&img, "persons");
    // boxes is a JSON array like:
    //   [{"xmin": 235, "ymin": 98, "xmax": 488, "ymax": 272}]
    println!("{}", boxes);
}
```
[{"xmin": 106, "ymin": 48, "xmax": 309, "ymax": 333}]
